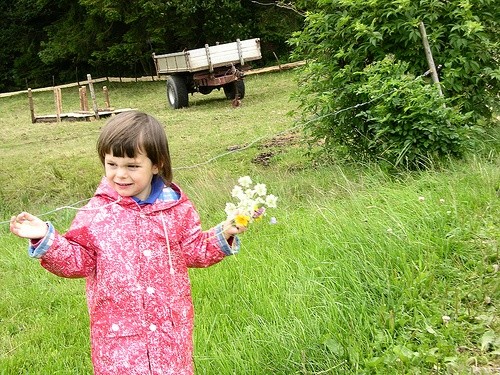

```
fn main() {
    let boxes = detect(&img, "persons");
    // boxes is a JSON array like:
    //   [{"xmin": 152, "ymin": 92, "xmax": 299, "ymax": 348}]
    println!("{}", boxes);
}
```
[{"xmin": 9, "ymin": 110, "xmax": 246, "ymax": 375}]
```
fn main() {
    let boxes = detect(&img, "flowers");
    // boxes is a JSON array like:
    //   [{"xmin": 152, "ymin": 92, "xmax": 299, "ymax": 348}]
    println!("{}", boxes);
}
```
[{"xmin": 224, "ymin": 176, "xmax": 278, "ymax": 226}]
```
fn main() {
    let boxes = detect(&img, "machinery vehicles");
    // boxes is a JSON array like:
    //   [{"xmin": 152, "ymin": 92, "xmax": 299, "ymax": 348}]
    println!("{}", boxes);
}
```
[{"xmin": 152, "ymin": 38, "xmax": 263, "ymax": 110}]
